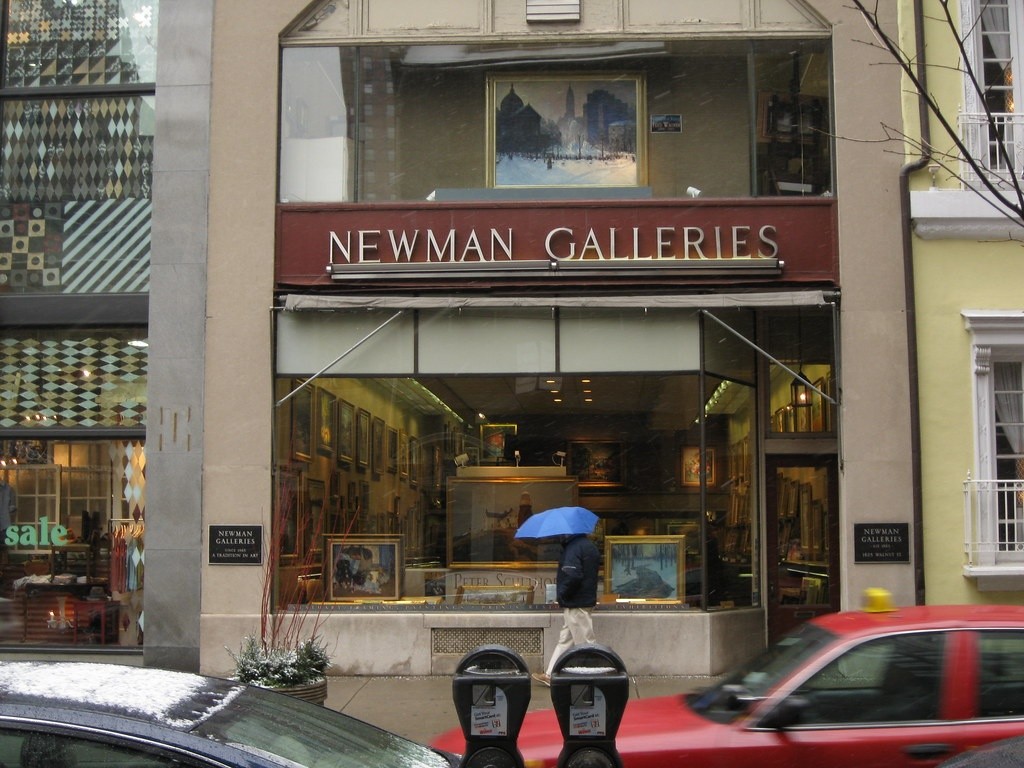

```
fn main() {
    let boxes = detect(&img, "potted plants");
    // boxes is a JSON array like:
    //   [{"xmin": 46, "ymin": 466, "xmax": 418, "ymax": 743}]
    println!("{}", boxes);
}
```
[{"xmin": 225, "ymin": 636, "xmax": 329, "ymax": 709}]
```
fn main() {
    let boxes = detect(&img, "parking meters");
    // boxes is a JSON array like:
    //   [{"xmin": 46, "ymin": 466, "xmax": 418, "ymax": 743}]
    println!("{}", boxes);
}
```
[
  {"xmin": 549, "ymin": 643, "xmax": 630, "ymax": 768},
  {"xmin": 451, "ymin": 642, "xmax": 532, "ymax": 768}
]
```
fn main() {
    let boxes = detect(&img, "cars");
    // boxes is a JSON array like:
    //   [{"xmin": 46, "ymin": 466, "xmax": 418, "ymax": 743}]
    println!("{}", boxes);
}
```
[
  {"xmin": 939, "ymin": 731, "xmax": 1024, "ymax": 768},
  {"xmin": 0, "ymin": 658, "xmax": 463, "ymax": 768},
  {"xmin": 405, "ymin": 568, "xmax": 557, "ymax": 605},
  {"xmin": 598, "ymin": 559, "xmax": 839, "ymax": 645},
  {"xmin": 425, "ymin": 587, "xmax": 1024, "ymax": 768},
  {"xmin": 48, "ymin": 596, "xmax": 120, "ymax": 650}
]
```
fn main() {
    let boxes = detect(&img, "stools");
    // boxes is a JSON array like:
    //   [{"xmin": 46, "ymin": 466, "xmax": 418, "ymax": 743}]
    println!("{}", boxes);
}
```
[
  {"xmin": 75, "ymin": 600, "xmax": 119, "ymax": 645},
  {"xmin": 49, "ymin": 542, "xmax": 92, "ymax": 584}
]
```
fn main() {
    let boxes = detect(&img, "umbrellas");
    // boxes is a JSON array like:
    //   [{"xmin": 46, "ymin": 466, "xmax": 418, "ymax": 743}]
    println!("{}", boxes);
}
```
[{"xmin": 513, "ymin": 505, "xmax": 600, "ymax": 540}]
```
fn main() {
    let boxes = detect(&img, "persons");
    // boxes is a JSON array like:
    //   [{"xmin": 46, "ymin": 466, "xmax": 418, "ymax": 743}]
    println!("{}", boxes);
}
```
[
  {"xmin": 531, "ymin": 534, "xmax": 602, "ymax": 686},
  {"xmin": 882, "ymin": 634, "xmax": 946, "ymax": 722}
]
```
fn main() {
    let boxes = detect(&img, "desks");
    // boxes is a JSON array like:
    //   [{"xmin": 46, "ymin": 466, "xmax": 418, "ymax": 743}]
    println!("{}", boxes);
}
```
[{"xmin": 21, "ymin": 578, "xmax": 108, "ymax": 643}]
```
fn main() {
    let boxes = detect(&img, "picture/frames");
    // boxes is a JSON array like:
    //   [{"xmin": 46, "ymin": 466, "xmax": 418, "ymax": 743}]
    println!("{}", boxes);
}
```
[
  {"xmin": 272, "ymin": 370, "xmax": 838, "ymax": 610},
  {"xmin": 756, "ymin": 90, "xmax": 823, "ymax": 146},
  {"xmin": 484, "ymin": 69, "xmax": 648, "ymax": 191}
]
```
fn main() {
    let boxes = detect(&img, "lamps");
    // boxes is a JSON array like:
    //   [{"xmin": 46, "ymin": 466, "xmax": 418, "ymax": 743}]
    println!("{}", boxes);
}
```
[
  {"xmin": 1004, "ymin": 68, "xmax": 1013, "ymax": 112},
  {"xmin": 790, "ymin": 308, "xmax": 813, "ymax": 407},
  {"xmin": 454, "ymin": 452, "xmax": 470, "ymax": 468}
]
